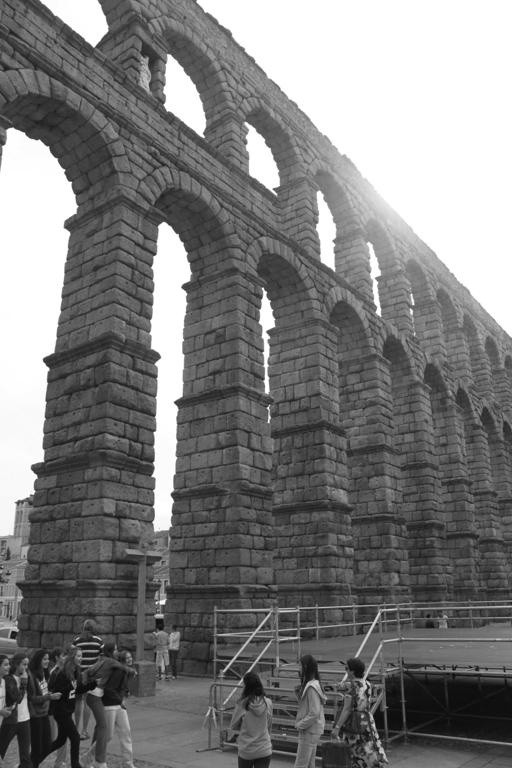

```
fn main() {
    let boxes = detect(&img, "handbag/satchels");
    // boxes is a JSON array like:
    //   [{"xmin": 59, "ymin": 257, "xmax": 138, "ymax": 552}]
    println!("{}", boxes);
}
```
[
  {"xmin": 77, "ymin": 676, "xmax": 97, "ymax": 695},
  {"xmin": 332, "ymin": 706, "xmax": 371, "ymax": 734},
  {"xmin": 321, "ymin": 741, "xmax": 351, "ymax": 768}
]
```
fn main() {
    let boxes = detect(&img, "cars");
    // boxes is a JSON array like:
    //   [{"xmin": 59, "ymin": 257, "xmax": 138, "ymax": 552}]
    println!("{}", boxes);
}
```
[{"xmin": 0, "ymin": 627, "xmax": 20, "ymax": 654}]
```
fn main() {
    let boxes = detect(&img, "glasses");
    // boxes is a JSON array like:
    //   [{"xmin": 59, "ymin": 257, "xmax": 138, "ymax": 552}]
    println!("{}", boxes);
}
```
[{"xmin": 345, "ymin": 666, "xmax": 349, "ymax": 670}]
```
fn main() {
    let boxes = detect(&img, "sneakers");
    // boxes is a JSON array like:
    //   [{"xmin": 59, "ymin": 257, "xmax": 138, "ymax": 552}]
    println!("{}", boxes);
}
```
[
  {"xmin": 119, "ymin": 760, "xmax": 135, "ymax": 768},
  {"xmin": 54, "ymin": 760, "xmax": 71, "ymax": 768},
  {"xmin": 92, "ymin": 759, "xmax": 107, "ymax": 768},
  {"xmin": 155, "ymin": 675, "xmax": 176, "ymax": 680},
  {"xmin": 79, "ymin": 729, "xmax": 89, "ymax": 740}
]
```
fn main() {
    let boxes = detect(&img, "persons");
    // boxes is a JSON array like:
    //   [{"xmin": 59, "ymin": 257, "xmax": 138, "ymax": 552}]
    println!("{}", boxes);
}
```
[
  {"xmin": 153, "ymin": 621, "xmax": 170, "ymax": 680},
  {"xmin": 0, "ymin": 653, "xmax": 12, "ymax": 728},
  {"xmin": 227, "ymin": 671, "xmax": 275, "ymax": 766},
  {"xmin": 425, "ymin": 612, "xmax": 434, "ymax": 628},
  {"xmin": 49, "ymin": 646, "xmax": 84, "ymax": 767},
  {"xmin": 79, "ymin": 647, "xmax": 137, "ymax": 767},
  {"xmin": 332, "ymin": 658, "xmax": 390, "ymax": 767},
  {"xmin": 85, "ymin": 640, "xmax": 138, "ymax": 767},
  {"xmin": 0, "ymin": 651, "xmax": 33, "ymax": 767},
  {"xmin": 50, "ymin": 655, "xmax": 67, "ymax": 767},
  {"xmin": 25, "ymin": 647, "xmax": 62, "ymax": 767},
  {"xmin": 72, "ymin": 617, "xmax": 105, "ymax": 738},
  {"xmin": 291, "ymin": 656, "xmax": 327, "ymax": 767},
  {"xmin": 437, "ymin": 609, "xmax": 449, "ymax": 628},
  {"xmin": 166, "ymin": 623, "xmax": 181, "ymax": 680}
]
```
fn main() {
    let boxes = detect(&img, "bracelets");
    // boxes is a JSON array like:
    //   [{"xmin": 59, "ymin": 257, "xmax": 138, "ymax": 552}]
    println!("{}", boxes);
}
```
[{"xmin": 335, "ymin": 724, "xmax": 341, "ymax": 729}]
